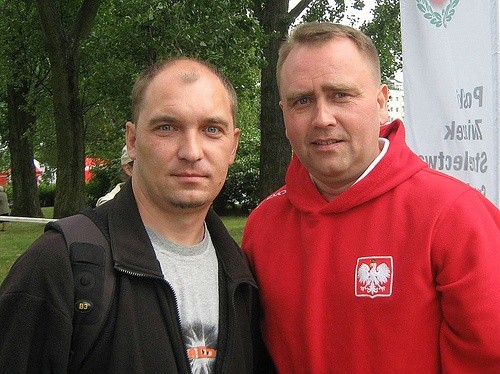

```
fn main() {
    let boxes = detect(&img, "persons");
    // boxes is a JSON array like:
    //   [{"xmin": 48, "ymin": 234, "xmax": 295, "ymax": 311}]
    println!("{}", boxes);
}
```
[
  {"xmin": 0, "ymin": 56, "xmax": 261, "ymax": 374},
  {"xmin": 244, "ymin": 22, "xmax": 500, "ymax": 374},
  {"xmin": 0, "ymin": 186, "xmax": 10, "ymax": 231},
  {"xmin": 94, "ymin": 145, "xmax": 134, "ymax": 207}
]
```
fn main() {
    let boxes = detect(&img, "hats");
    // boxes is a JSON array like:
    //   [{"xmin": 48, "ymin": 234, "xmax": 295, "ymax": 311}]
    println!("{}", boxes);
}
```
[{"xmin": 121, "ymin": 145, "xmax": 133, "ymax": 167}]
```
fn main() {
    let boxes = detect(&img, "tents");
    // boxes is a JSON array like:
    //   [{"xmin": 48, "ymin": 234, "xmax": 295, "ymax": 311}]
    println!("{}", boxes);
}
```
[{"xmin": 0, "ymin": 154, "xmax": 104, "ymax": 191}]
